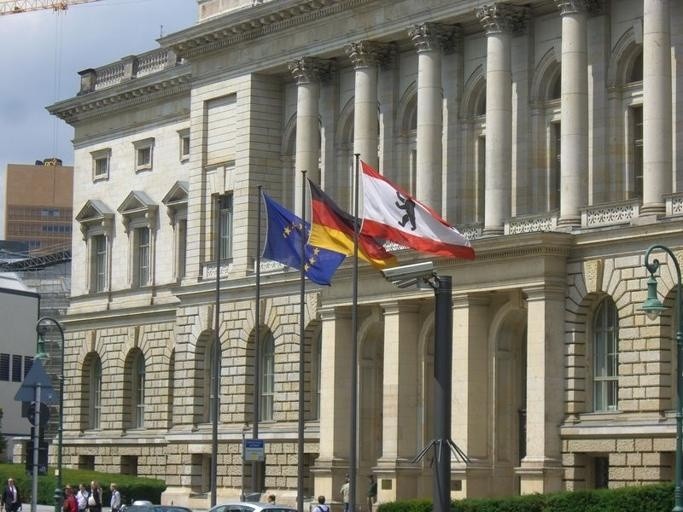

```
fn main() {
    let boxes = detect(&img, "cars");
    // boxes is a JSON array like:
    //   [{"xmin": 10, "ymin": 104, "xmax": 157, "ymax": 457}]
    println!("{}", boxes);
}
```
[
  {"xmin": 210, "ymin": 501, "xmax": 297, "ymax": 512},
  {"xmin": 115, "ymin": 504, "xmax": 190, "ymax": 512}
]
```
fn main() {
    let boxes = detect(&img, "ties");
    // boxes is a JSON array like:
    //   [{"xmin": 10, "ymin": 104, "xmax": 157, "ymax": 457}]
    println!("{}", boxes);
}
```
[{"xmin": 11, "ymin": 487, "xmax": 13, "ymax": 501}]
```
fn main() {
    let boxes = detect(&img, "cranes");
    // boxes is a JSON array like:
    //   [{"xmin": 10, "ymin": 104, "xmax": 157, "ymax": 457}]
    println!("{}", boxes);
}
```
[
  {"xmin": 1, "ymin": 0, "xmax": 99, "ymax": 16},
  {"xmin": 1, "ymin": 245, "xmax": 71, "ymax": 272}
]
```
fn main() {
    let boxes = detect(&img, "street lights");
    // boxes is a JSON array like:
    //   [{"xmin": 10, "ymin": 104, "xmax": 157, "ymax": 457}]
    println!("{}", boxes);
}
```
[
  {"xmin": 33, "ymin": 316, "xmax": 64, "ymax": 511},
  {"xmin": 637, "ymin": 244, "xmax": 683, "ymax": 511}
]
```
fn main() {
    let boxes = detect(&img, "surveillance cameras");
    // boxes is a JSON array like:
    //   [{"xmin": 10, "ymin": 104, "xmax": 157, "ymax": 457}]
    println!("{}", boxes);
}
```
[{"xmin": 379, "ymin": 260, "xmax": 436, "ymax": 289}]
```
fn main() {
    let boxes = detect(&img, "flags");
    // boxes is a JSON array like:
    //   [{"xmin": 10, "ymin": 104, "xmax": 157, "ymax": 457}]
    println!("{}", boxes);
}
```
[
  {"xmin": 258, "ymin": 191, "xmax": 345, "ymax": 285},
  {"xmin": 359, "ymin": 159, "xmax": 476, "ymax": 263},
  {"xmin": 306, "ymin": 178, "xmax": 397, "ymax": 269}
]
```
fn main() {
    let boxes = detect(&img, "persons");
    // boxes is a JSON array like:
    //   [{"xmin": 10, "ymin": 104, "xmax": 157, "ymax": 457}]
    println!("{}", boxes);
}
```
[
  {"xmin": 0, "ymin": 478, "xmax": 22, "ymax": 511},
  {"xmin": 366, "ymin": 474, "xmax": 377, "ymax": 512},
  {"xmin": 63, "ymin": 480, "xmax": 121, "ymax": 512},
  {"xmin": 268, "ymin": 494, "xmax": 275, "ymax": 504},
  {"xmin": 311, "ymin": 496, "xmax": 329, "ymax": 512},
  {"xmin": 340, "ymin": 478, "xmax": 351, "ymax": 512}
]
